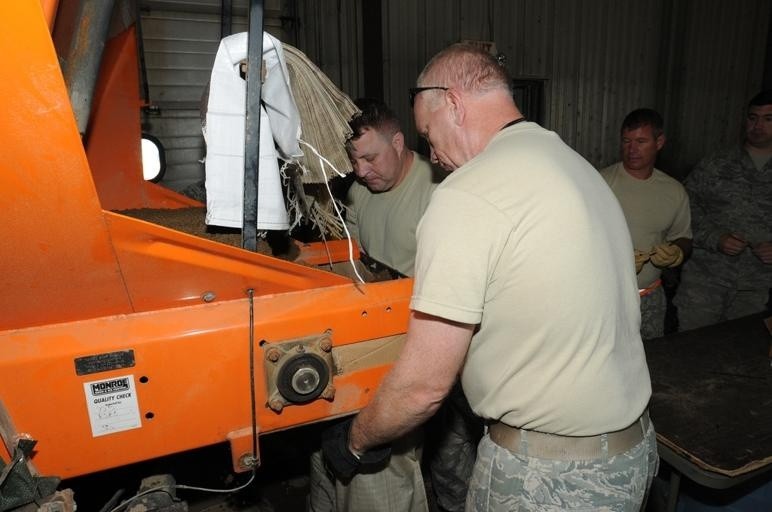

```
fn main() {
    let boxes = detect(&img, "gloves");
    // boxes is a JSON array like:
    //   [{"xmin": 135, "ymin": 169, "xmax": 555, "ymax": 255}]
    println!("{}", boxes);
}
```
[{"xmin": 321, "ymin": 416, "xmax": 394, "ymax": 486}]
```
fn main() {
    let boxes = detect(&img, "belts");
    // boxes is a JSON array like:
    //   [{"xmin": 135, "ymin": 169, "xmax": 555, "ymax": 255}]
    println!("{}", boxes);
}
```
[
  {"xmin": 489, "ymin": 405, "xmax": 650, "ymax": 463},
  {"xmin": 638, "ymin": 277, "xmax": 661, "ymax": 298}
]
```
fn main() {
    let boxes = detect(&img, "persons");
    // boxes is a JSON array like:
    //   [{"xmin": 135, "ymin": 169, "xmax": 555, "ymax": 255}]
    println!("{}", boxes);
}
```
[
  {"xmin": 324, "ymin": 41, "xmax": 662, "ymax": 512},
  {"xmin": 596, "ymin": 103, "xmax": 694, "ymax": 345},
  {"xmin": 663, "ymin": 92, "xmax": 771, "ymax": 338},
  {"xmin": 339, "ymin": 100, "xmax": 483, "ymax": 512}
]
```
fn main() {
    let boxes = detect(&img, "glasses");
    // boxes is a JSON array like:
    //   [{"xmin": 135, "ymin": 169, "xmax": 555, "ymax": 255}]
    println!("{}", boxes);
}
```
[{"xmin": 407, "ymin": 86, "xmax": 448, "ymax": 107}]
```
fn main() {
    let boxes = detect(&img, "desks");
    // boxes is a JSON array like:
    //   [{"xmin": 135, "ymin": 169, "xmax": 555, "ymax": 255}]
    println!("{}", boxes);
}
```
[{"xmin": 642, "ymin": 310, "xmax": 771, "ymax": 511}]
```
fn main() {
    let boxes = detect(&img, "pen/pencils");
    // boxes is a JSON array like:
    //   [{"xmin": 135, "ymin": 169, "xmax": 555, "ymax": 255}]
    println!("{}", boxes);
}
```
[
  {"xmin": 748, "ymin": 241, "xmax": 753, "ymax": 249},
  {"xmin": 643, "ymin": 240, "xmax": 672, "ymax": 256}
]
